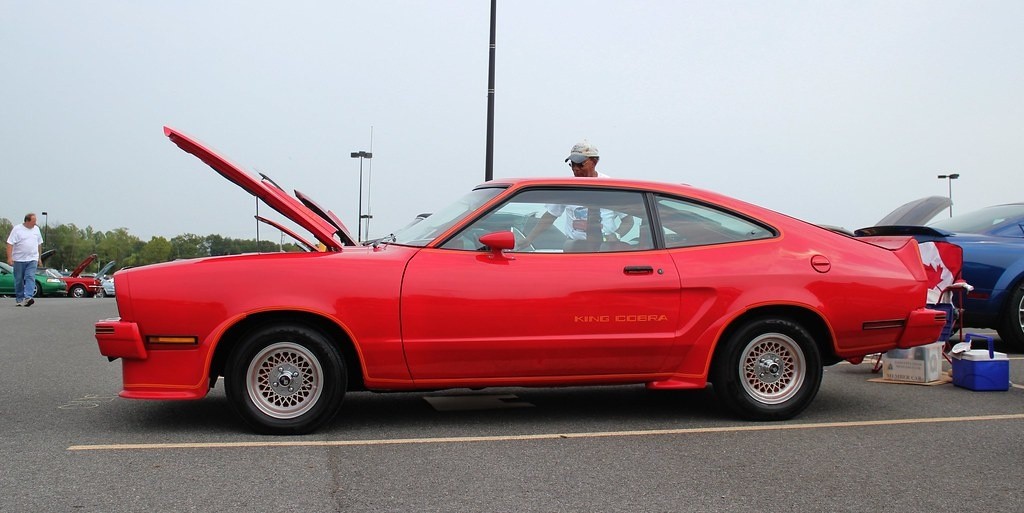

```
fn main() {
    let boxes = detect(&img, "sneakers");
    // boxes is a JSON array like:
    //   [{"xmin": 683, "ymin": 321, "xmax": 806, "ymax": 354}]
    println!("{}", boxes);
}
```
[
  {"xmin": 23, "ymin": 298, "xmax": 35, "ymax": 307},
  {"xmin": 16, "ymin": 301, "xmax": 23, "ymax": 306}
]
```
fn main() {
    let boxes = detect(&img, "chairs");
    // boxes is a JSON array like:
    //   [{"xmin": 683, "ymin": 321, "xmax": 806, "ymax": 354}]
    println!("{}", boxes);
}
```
[{"xmin": 563, "ymin": 230, "xmax": 712, "ymax": 252}]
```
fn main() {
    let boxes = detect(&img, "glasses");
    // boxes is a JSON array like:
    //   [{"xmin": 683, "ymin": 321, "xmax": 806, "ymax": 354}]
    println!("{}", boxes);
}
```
[{"xmin": 569, "ymin": 159, "xmax": 590, "ymax": 167}]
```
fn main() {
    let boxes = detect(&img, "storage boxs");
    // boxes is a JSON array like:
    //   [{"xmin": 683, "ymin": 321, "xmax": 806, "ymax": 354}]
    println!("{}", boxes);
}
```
[
  {"xmin": 952, "ymin": 333, "xmax": 1009, "ymax": 390},
  {"xmin": 881, "ymin": 341, "xmax": 945, "ymax": 382}
]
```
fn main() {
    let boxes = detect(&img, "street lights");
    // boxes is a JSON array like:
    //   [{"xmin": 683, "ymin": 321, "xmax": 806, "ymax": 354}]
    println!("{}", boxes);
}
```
[
  {"xmin": 938, "ymin": 173, "xmax": 959, "ymax": 218},
  {"xmin": 41, "ymin": 211, "xmax": 49, "ymax": 252},
  {"xmin": 351, "ymin": 150, "xmax": 373, "ymax": 239}
]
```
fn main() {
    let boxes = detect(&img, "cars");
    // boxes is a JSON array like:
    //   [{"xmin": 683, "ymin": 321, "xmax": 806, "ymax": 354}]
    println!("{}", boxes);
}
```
[
  {"xmin": 1, "ymin": 249, "xmax": 59, "ymax": 300},
  {"xmin": 929, "ymin": 201, "xmax": 1024, "ymax": 351},
  {"xmin": 67, "ymin": 259, "xmax": 114, "ymax": 298},
  {"xmin": 61, "ymin": 252, "xmax": 102, "ymax": 299},
  {"xmin": 89, "ymin": 122, "xmax": 950, "ymax": 434},
  {"xmin": 36, "ymin": 265, "xmax": 63, "ymax": 279}
]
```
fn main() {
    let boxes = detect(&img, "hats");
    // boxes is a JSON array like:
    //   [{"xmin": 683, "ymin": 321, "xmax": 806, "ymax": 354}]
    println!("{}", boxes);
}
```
[{"xmin": 565, "ymin": 143, "xmax": 599, "ymax": 164}]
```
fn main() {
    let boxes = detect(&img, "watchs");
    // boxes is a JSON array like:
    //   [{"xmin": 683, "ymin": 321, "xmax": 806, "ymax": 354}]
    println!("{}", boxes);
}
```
[{"xmin": 611, "ymin": 230, "xmax": 621, "ymax": 241}]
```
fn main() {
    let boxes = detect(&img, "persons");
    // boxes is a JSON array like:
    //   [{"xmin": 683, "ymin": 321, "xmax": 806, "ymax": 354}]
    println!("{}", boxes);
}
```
[
  {"xmin": 517, "ymin": 139, "xmax": 634, "ymax": 257},
  {"xmin": 6, "ymin": 213, "xmax": 43, "ymax": 307}
]
